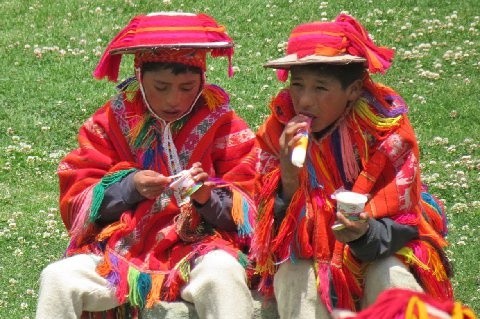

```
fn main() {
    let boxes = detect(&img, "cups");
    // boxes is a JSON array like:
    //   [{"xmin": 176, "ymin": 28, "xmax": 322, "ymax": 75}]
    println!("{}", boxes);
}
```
[
  {"xmin": 336, "ymin": 193, "xmax": 368, "ymax": 221},
  {"xmin": 169, "ymin": 167, "xmax": 203, "ymax": 199}
]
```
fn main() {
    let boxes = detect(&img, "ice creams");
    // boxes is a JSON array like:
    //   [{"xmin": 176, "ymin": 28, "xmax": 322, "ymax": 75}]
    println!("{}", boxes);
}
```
[
  {"xmin": 168, "ymin": 167, "xmax": 204, "ymax": 200},
  {"xmin": 334, "ymin": 192, "xmax": 368, "ymax": 220}
]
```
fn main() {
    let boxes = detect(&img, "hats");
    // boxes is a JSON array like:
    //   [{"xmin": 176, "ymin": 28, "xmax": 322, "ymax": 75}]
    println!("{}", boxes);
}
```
[
  {"xmin": 92, "ymin": 11, "xmax": 234, "ymax": 82},
  {"xmin": 263, "ymin": 13, "xmax": 395, "ymax": 83}
]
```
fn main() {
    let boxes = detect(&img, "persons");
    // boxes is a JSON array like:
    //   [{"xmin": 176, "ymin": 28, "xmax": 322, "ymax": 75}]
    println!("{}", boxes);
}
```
[
  {"xmin": 251, "ymin": 12, "xmax": 454, "ymax": 319},
  {"xmin": 33, "ymin": 11, "xmax": 256, "ymax": 319}
]
